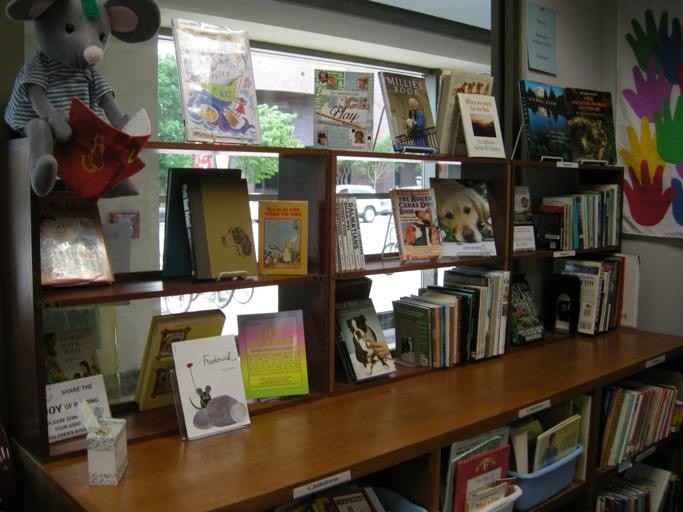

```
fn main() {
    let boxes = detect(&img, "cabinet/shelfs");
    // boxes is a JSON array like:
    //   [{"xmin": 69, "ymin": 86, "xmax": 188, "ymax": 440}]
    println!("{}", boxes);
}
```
[{"xmin": 0, "ymin": 137, "xmax": 683, "ymax": 512}]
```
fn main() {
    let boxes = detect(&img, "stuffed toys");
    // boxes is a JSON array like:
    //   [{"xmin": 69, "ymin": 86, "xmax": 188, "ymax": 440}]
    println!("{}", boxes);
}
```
[{"xmin": 4, "ymin": 0, "xmax": 164, "ymax": 199}]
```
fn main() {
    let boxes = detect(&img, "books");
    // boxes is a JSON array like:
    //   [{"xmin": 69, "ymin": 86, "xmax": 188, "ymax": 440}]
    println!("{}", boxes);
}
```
[
  {"xmin": 456, "ymin": 92, "xmax": 507, "ymax": 161},
  {"xmin": 43, "ymin": 305, "xmax": 118, "ymax": 461},
  {"xmin": 335, "ymin": 277, "xmax": 396, "ymax": 384},
  {"xmin": 379, "ymin": 71, "xmax": 439, "ymax": 158},
  {"xmin": 312, "ymin": 486, "xmax": 385, "ymax": 512},
  {"xmin": 335, "ymin": 196, "xmax": 366, "ymax": 272},
  {"xmin": 566, "ymin": 86, "xmax": 618, "ymax": 166},
  {"xmin": 388, "ymin": 176, "xmax": 619, "ymax": 369},
  {"xmin": 517, "ymin": 78, "xmax": 572, "ymax": 162},
  {"xmin": 134, "ymin": 309, "xmax": 309, "ymax": 441},
  {"xmin": 309, "ymin": 68, "xmax": 376, "ymax": 152},
  {"xmin": 562, "ymin": 380, "xmax": 681, "ymax": 511},
  {"xmin": 171, "ymin": 15, "xmax": 261, "ymax": 145},
  {"xmin": 437, "ymin": 68, "xmax": 497, "ymax": 158},
  {"xmin": 30, "ymin": 187, "xmax": 118, "ymax": 291},
  {"xmin": 443, "ymin": 413, "xmax": 582, "ymax": 512},
  {"xmin": 162, "ymin": 167, "xmax": 308, "ymax": 280}
]
